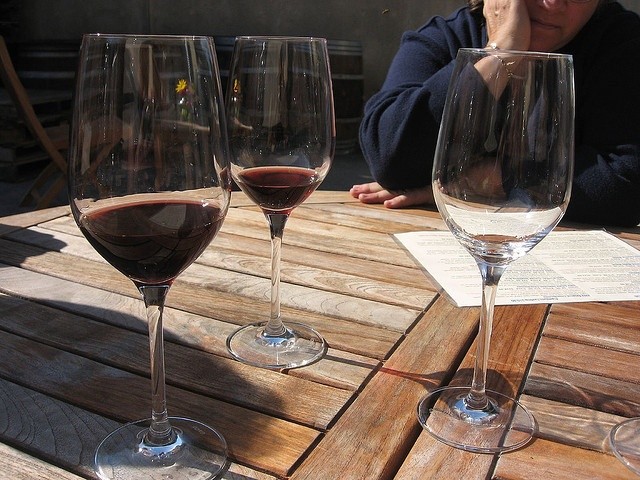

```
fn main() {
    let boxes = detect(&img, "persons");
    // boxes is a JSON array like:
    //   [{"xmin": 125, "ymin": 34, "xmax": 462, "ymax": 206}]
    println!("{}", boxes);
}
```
[{"xmin": 349, "ymin": 0, "xmax": 637, "ymax": 225}]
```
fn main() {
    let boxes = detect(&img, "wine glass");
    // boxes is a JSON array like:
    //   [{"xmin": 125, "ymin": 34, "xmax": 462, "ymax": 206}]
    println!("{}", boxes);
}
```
[
  {"xmin": 416, "ymin": 46, "xmax": 576, "ymax": 456},
  {"xmin": 226, "ymin": 33, "xmax": 337, "ymax": 370},
  {"xmin": 69, "ymin": 34, "xmax": 232, "ymax": 480}
]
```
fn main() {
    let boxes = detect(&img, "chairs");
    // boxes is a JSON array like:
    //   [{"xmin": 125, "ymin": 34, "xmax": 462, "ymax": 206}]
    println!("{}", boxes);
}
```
[{"xmin": 0, "ymin": 34, "xmax": 135, "ymax": 210}]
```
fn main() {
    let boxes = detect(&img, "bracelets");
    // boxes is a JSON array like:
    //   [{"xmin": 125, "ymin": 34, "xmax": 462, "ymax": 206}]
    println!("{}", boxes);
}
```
[{"xmin": 485, "ymin": 40, "xmax": 517, "ymax": 80}]
